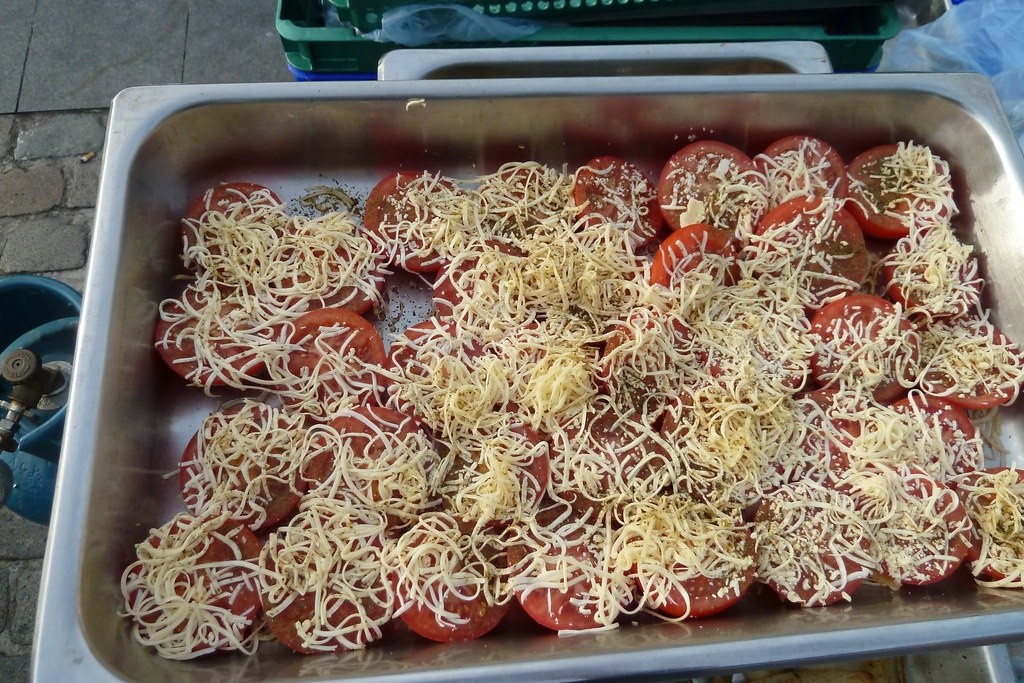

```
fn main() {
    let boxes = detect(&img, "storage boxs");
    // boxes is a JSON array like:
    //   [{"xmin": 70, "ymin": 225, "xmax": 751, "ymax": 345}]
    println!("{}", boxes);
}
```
[{"xmin": 273, "ymin": 0, "xmax": 904, "ymax": 75}]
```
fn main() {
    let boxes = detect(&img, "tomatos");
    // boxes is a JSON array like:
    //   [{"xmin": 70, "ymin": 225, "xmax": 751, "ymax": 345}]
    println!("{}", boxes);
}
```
[{"xmin": 123, "ymin": 137, "xmax": 1023, "ymax": 652}]
